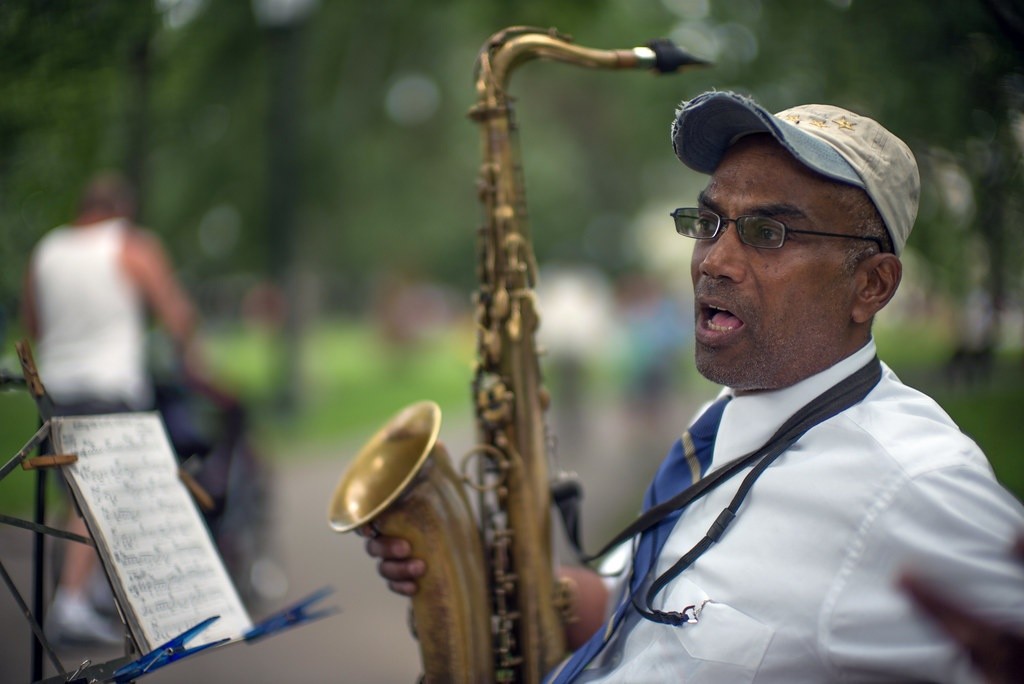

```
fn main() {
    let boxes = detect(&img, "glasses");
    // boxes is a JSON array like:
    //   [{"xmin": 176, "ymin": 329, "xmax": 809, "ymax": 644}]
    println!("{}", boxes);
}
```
[{"xmin": 670, "ymin": 207, "xmax": 886, "ymax": 253}]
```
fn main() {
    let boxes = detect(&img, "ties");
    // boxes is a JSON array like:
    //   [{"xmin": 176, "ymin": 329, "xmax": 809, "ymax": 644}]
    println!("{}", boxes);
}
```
[{"xmin": 541, "ymin": 396, "xmax": 732, "ymax": 684}]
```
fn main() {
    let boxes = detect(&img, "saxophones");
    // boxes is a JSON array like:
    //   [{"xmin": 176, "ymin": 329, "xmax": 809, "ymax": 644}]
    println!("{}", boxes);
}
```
[{"xmin": 328, "ymin": 24, "xmax": 718, "ymax": 684}]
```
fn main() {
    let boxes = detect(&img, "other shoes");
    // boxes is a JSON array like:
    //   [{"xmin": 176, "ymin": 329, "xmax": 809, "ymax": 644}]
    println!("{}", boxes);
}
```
[{"xmin": 49, "ymin": 594, "xmax": 116, "ymax": 646}]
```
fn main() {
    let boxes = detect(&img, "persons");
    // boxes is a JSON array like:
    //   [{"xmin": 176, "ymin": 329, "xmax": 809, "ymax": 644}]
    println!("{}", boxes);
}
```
[
  {"xmin": 27, "ymin": 170, "xmax": 245, "ymax": 650},
  {"xmin": 364, "ymin": 92, "xmax": 1024, "ymax": 684}
]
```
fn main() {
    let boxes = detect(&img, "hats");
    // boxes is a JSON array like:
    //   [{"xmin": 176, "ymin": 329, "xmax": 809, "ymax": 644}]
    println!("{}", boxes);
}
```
[{"xmin": 671, "ymin": 91, "xmax": 919, "ymax": 257}]
